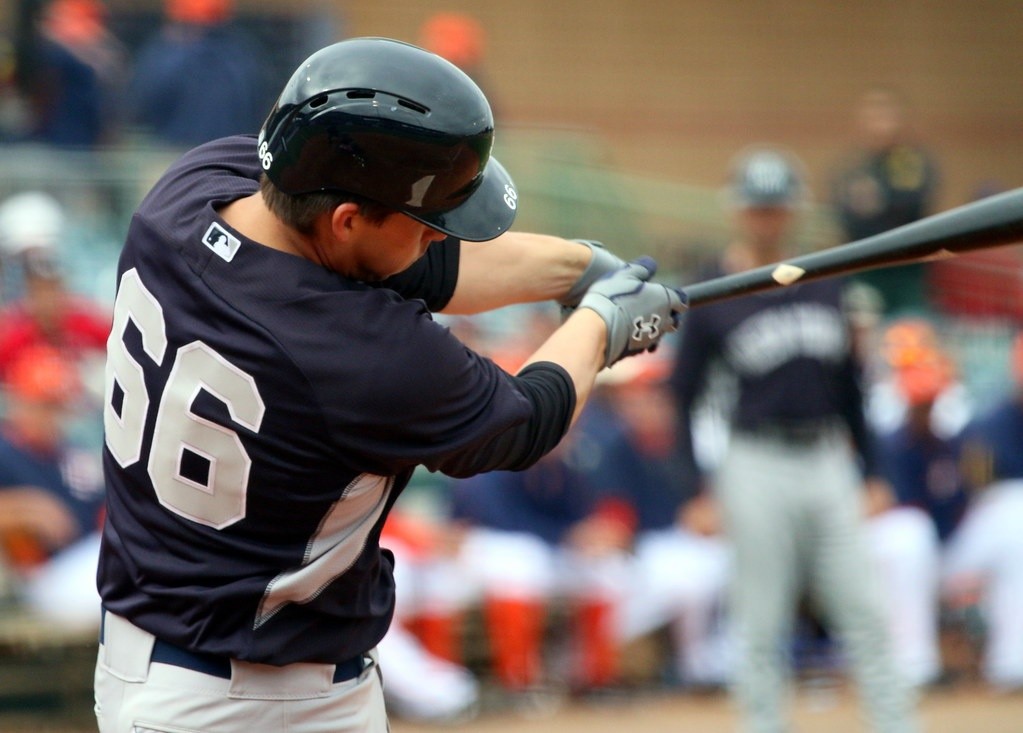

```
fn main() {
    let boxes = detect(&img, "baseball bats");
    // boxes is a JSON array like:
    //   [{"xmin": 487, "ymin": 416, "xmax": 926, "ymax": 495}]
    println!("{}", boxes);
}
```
[{"xmin": 563, "ymin": 185, "xmax": 1023, "ymax": 326}]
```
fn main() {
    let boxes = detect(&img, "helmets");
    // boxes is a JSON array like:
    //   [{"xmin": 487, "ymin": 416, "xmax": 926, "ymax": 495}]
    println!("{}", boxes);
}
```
[{"xmin": 257, "ymin": 36, "xmax": 519, "ymax": 242}]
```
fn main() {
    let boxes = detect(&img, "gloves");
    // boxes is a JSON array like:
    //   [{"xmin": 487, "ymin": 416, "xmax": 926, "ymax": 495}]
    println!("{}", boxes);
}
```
[
  {"xmin": 558, "ymin": 239, "xmax": 628, "ymax": 326},
  {"xmin": 578, "ymin": 255, "xmax": 688, "ymax": 372}
]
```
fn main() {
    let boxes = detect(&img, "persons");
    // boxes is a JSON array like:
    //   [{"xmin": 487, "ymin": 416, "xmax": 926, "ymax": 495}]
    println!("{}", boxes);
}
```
[
  {"xmin": 88, "ymin": 38, "xmax": 686, "ymax": 733},
  {"xmin": 0, "ymin": 2, "xmax": 1022, "ymax": 732}
]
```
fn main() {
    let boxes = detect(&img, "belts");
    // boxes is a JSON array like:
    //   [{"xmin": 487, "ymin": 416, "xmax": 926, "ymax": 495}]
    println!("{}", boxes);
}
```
[{"xmin": 98, "ymin": 604, "xmax": 374, "ymax": 684}]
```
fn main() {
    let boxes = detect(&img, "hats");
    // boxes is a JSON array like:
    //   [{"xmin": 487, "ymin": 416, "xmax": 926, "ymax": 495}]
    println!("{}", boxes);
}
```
[
  {"xmin": 14, "ymin": 343, "xmax": 80, "ymax": 403},
  {"xmin": 0, "ymin": 191, "xmax": 64, "ymax": 254},
  {"xmin": 735, "ymin": 147, "xmax": 795, "ymax": 208}
]
